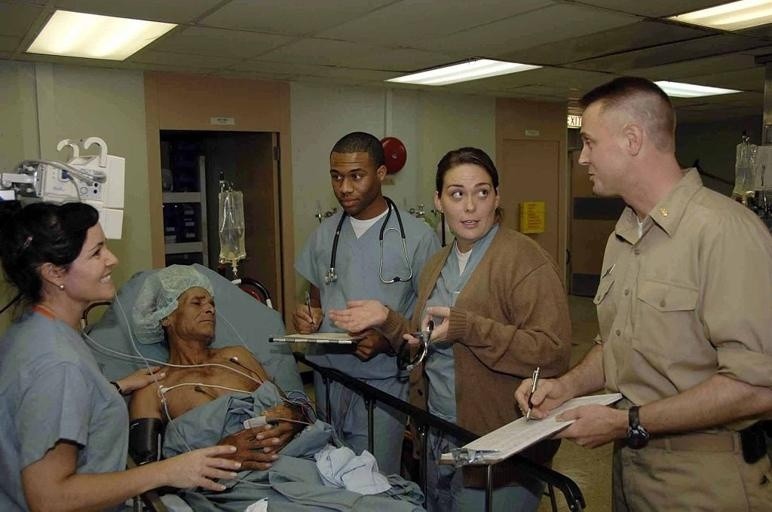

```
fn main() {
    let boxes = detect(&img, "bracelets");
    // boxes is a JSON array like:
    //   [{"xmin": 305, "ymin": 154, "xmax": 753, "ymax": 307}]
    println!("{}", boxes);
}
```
[{"xmin": 109, "ymin": 379, "xmax": 122, "ymax": 397}]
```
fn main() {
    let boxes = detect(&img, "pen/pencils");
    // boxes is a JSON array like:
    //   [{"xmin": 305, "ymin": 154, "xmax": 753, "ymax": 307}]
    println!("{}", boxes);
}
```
[
  {"xmin": 304, "ymin": 291, "xmax": 314, "ymax": 327},
  {"xmin": 526, "ymin": 366, "xmax": 540, "ymax": 419}
]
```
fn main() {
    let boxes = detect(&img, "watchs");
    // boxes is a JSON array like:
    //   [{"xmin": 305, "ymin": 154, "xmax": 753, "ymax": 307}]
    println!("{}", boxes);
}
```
[{"xmin": 627, "ymin": 403, "xmax": 649, "ymax": 451}]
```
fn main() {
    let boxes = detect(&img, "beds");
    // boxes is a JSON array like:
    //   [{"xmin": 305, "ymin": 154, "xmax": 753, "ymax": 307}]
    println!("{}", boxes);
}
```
[{"xmin": 83, "ymin": 263, "xmax": 586, "ymax": 512}]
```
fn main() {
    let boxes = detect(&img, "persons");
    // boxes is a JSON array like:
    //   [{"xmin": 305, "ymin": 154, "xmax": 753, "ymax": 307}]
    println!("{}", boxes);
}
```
[
  {"xmin": 0, "ymin": 199, "xmax": 242, "ymax": 512},
  {"xmin": 126, "ymin": 262, "xmax": 317, "ymax": 496},
  {"xmin": 512, "ymin": 73, "xmax": 772, "ymax": 511},
  {"xmin": 291, "ymin": 130, "xmax": 442, "ymax": 482},
  {"xmin": 326, "ymin": 144, "xmax": 574, "ymax": 512}
]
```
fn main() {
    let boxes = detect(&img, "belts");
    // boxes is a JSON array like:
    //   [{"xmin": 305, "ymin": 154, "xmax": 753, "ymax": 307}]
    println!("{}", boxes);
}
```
[{"xmin": 649, "ymin": 431, "xmax": 742, "ymax": 453}]
